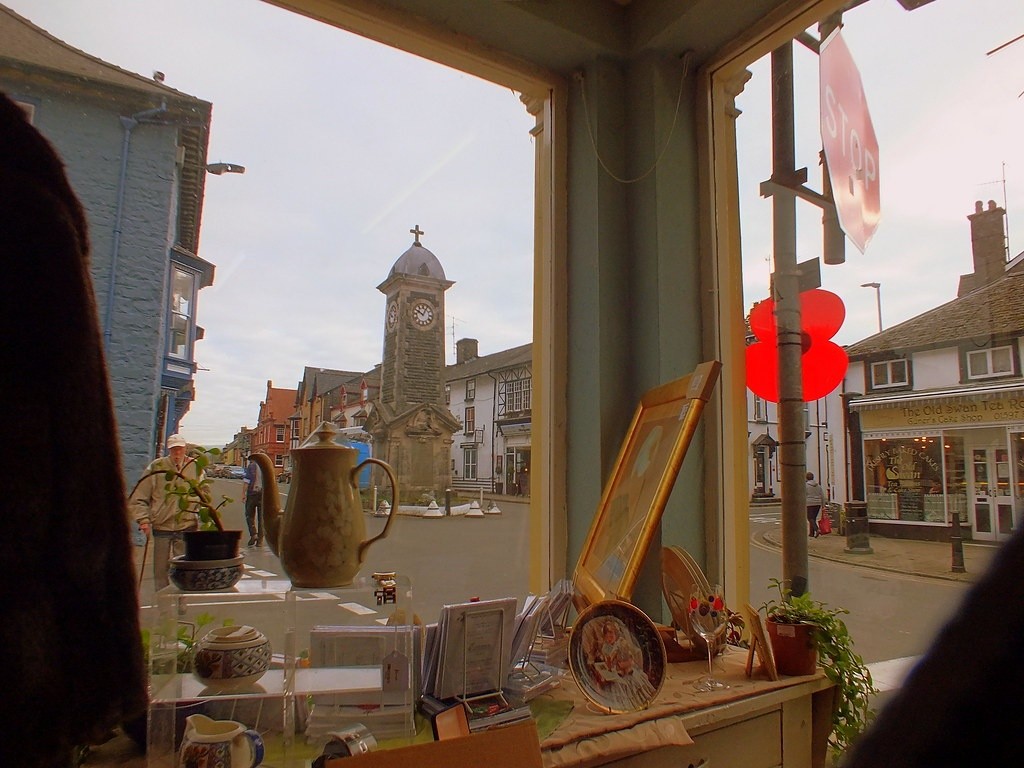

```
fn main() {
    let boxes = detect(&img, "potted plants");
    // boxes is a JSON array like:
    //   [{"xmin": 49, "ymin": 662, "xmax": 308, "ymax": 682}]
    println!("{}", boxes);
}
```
[
  {"xmin": 760, "ymin": 577, "xmax": 880, "ymax": 759},
  {"xmin": 127, "ymin": 447, "xmax": 244, "ymax": 591}
]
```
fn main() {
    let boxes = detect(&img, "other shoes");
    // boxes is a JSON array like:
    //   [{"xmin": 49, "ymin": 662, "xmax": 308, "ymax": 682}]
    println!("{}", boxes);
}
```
[{"xmin": 248, "ymin": 536, "xmax": 257, "ymax": 547}]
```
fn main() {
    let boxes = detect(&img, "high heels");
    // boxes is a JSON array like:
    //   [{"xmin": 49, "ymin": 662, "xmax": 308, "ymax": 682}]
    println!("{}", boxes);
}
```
[{"xmin": 814, "ymin": 530, "xmax": 822, "ymax": 538}]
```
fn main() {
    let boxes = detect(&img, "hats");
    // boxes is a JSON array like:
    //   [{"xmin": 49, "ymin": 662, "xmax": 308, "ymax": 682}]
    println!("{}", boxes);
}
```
[{"xmin": 167, "ymin": 434, "xmax": 187, "ymax": 447}]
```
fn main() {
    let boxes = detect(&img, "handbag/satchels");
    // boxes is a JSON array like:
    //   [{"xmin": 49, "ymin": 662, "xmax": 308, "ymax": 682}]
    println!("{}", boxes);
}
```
[{"xmin": 818, "ymin": 508, "xmax": 831, "ymax": 535}]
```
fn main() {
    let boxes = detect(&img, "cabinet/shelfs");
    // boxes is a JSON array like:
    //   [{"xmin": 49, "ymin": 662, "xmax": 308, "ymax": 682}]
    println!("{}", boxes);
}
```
[{"xmin": 142, "ymin": 579, "xmax": 835, "ymax": 768}]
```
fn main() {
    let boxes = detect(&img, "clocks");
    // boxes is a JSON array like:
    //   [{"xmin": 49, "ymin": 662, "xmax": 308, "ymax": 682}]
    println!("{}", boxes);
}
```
[
  {"xmin": 386, "ymin": 300, "xmax": 399, "ymax": 332},
  {"xmin": 410, "ymin": 298, "xmax": 438, "ymax": 330}
]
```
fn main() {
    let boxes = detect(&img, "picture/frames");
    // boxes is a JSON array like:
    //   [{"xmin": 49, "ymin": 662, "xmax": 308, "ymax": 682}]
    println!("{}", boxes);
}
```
[
  {"xmin": 572, "ymin": 363, "xmax": 720, "ymax": 602},
  {"xmin": 744, "ymin": 603, "xmax": 780, "ymax": 682}
]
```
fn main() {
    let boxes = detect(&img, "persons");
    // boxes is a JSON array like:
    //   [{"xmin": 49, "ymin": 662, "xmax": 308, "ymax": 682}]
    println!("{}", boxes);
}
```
[
  {"xmin": 242, "ymin": 448, "xmax": 265, "ymax": 547},
  {"xmin": 131, "ymin": 434, "xmax": 212, "ymax": 591},
  {"xmin": 806, "ymin": 472, "xmax": 830, "ymax": 539}
]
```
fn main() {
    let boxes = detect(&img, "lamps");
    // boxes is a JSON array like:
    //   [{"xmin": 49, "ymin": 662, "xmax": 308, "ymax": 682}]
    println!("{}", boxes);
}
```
[{"xmin": 184, "ymin": 161, "xmax": 245, "ymax": 174}]
